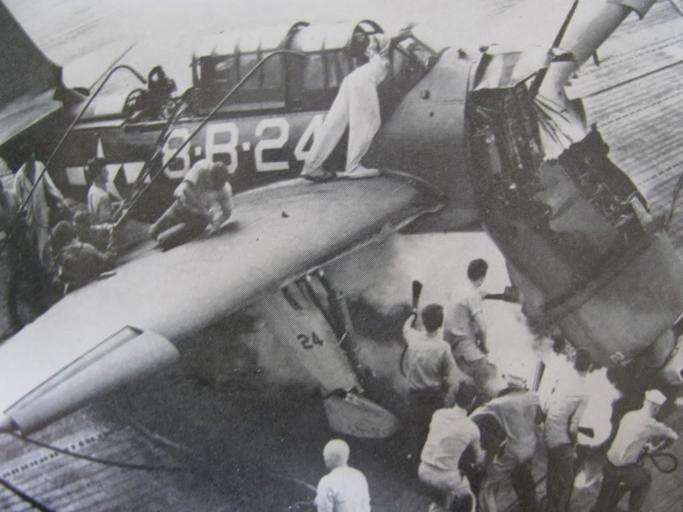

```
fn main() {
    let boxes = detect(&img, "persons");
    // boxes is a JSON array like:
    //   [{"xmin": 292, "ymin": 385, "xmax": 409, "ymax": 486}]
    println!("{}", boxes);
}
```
[
  {"xmin": 0, "ymin": 146, "xmax": 128, "ymax": 300},
  {"xmin": 300, "ymin": 23, "xmax": 418, "ymax": 180},
  {"xmin": 313, "ymin": 437, "xmax": 372, "ymax": 512},
  {"xmin": 148, "ymin": 158, "xmax": 234, "ymax": 249},
  {"xmin": 401, "ymin": 259, "xmax": 678, "ymax": 511}
]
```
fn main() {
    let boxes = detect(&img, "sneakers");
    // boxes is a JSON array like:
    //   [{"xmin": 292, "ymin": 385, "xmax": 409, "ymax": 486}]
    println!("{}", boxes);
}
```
[
  {"xmin": 301, "ymin": 167, "xmax": 331, "ymax": 181},
  {"xmin": 336, "ymin": 165, "xmax": 378, "ymax": 180}
]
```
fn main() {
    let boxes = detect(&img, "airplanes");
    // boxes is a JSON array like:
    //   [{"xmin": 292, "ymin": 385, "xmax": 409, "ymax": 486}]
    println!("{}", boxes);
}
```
[{"xmin": 0, "ymin": 1, "xmax": 683, "ymax": 438}]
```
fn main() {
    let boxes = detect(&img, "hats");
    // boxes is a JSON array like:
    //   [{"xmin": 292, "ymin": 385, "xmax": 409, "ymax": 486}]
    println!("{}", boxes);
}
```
[{"xmin": 645, "ymin": 389, "xmax": 667, "ymax": 406}]
